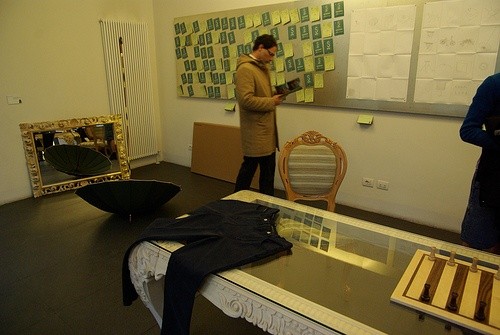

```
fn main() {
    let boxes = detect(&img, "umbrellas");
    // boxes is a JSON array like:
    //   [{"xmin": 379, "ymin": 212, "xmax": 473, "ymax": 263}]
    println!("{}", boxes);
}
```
[
  {"xmin": 44, "ymin": 144, "xmax": 111, "ymax": 177},
  {"xmin": 74, "ymin": 37, "xmax": 182, "ymax": 222}
]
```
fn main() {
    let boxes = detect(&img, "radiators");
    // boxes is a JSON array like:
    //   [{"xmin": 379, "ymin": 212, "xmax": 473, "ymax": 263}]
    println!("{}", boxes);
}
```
[{"xmin": 98, "ymin": 18, "xmax": 161, "ymax": 164}]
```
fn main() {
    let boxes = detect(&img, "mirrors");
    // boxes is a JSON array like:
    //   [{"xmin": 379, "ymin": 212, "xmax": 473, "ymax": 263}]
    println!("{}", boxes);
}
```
[{"xmin": 20, "ymin": 115, "xmax": 131, "ymax": 199}]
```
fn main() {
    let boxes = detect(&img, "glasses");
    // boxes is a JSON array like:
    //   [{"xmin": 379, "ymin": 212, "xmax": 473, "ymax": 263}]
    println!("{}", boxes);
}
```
[{"xmin": 266, "ymin": 48, "xmax": 275, "ymax": 57}]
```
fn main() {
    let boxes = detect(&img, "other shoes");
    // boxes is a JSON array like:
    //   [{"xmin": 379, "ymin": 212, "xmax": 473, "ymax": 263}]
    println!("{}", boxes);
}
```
[{"xmin": 109, "ymin": 156, "xmax": 117, "ymax": 160}]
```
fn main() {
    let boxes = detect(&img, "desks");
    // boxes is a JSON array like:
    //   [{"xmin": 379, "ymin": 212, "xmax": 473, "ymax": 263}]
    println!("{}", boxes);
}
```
[
  {"xmin": 35, "ymin": 132, "xmax": 75, "ymax": 148},
  {"xmin": 126, "ymin": 188, "xmax": 500, "ymax": 335}
]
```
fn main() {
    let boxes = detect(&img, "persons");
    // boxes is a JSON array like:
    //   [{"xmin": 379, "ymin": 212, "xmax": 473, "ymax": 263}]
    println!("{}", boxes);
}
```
[
  {"xmin": 459, "ymin": 73, "xmax": 500, "ymax": 251},
  {"xmin": 234, "ymin": 34, "xmax": 286, "ymax": 195}
]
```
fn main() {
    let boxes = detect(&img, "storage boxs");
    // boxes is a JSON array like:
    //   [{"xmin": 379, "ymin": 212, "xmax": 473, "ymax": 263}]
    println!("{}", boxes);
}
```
[{"xmin": 190, "ymin": 122, "xmax": 260, "ymax": 189}]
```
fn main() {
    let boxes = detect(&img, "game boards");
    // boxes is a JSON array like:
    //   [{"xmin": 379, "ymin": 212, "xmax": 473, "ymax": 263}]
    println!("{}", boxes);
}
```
[{"xmin": 391, "ymin": 248, "xmax": 500, "ymax": 335}]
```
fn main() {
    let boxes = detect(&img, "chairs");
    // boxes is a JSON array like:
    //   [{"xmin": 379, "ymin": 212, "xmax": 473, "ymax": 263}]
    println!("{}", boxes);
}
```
[
  {"xmin": 69, "ymin": 125, "xmax": 109, "ymax": 156},
  {"xmin": 278, "ymin": 130, "xmax": 350, "ymax": 215}
]
